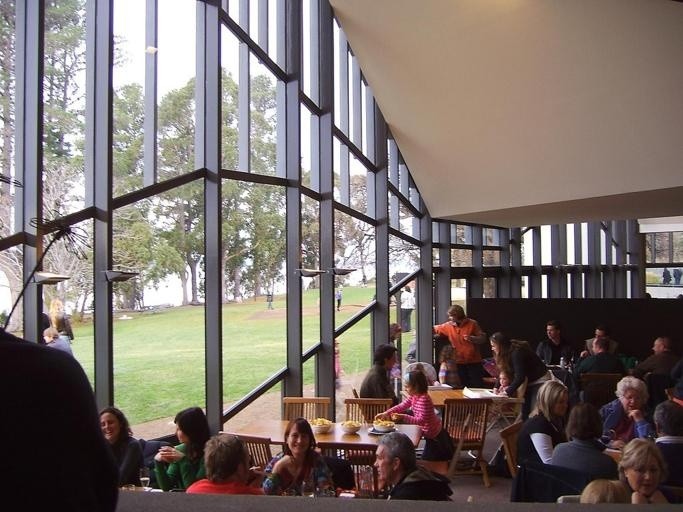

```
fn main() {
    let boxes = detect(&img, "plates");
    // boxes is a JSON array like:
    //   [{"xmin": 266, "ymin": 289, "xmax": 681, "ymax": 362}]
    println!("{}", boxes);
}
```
[{"xmin": 368, "ymin": 427, "xmax": 395, "ymax": 439}]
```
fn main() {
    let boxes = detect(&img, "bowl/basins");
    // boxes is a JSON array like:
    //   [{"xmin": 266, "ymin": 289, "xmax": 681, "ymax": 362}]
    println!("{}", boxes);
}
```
[
  {"xmin": 314, "ymin": 424, "xmax": 331, "ymax": 434},
  {"xmin": 341, "ymin": 426, "xmax": 361, "ymax": 435},
  {"xmin": 373, "ymin": 425, "xmax": 394, "ymax": 432}
]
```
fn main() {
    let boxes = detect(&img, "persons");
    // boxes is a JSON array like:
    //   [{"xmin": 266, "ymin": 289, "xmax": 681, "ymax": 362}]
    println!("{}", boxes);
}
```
[
  {"xmin": 477, "ymin": 368, "xmax": 517, "ymax": 424},
  {"xmin": 0, "ymin": 326, "xmax": 121, "ymax": 512},
  {"xmin": 412, "ymin": 304, "xmax": 487, "ymax": 388},
  {"xmin": 401, "ymin": 285, "xmax": 416, "ymax": 332},
  {"xmin": 374, "ymin": 371, "xmax": 455, "ymax": 476},
  {"xmin": 598, "ymin": 376, "xmax": 658, "ymax": 449},
  {"xmin": 49, "ymin": 296, "xmax": 75, "ymax": 345},
  {"xmin": 412, "ymin": 363, "xmax": 439, "ymax": 386},
  {"xmin": 536, "ymin": 319, "xmax": 573, "ymax": 365},
  {"xmin": 652, "ymin": 399, "xmax": 682, "ymax": 486},
  {"xmin": 390, "ymin": 322, "xmax": 402, "ymax": 392},
  {"xmin": 336, "ymin": 290, "xmax": 343, "ymax": 312},
  {"xmin": 187, "ymin": 433, "xmax": 266, "ymax": 494},
  {"xmin": 582, "ymin": 327, "xmax": 620, "ymax": 354},
  {"xmin": 628, "ymin": 335, "xmax": 680, "ymax": 412},
  {"xmin": 618, "ymin": 437, "xmax": 671, "ymax": 504},
  {"xmin": 153, "ymin": 407, "xmax": 212, "ymax": 491},
  {"xmin": 99, "ymin": 408, "xmax": 146, "ymax": 487},
  {"xmin": 517, "ymin": 380, "xmax": 621, "ymax": 474},
  {"xmin": 359, "ymin": 343, "xmax": 413, "ymax": 423},
  {"xmin": 550, "ymin": 403, "xmax": 619, "ymax": 480},
  {"xmin": 265, "ymin": 286, "xmax": 275, "ymax": 311},
  {"xmin": 332, "ymin": 339, "xmax": 340, "ymax": 390},
  {"xmin": 42, "ymin": 328, "xmax": 74, "ymax": 358},
  {"xmin": 373, "ymin": 432, "xmax": 453, "ymax": 501},
  {"xmin": 670, "ymin": 381, "xmax": 683, "ymax": 406},
  {"xmin": 674, "ymin": 269, "xmax": 682, "ymax": 284},
  {"xmin": 491, "ymin": 332, "xmax": 552, "ymax": 424},
  {"xmin": 40, "ymin": 297, "xmax": 53, "ymax": 344},
  {"xmin": 576, "ymin": 336, "xmax": 626, "ymax": 404},
  {"xmin": 662, "ymin": 268, "xmax": 672, "ymax": 283},
  {"xmin": 262, "ymin": 417, "xmax": 336, "ymax": 496},
  {"xmin": 579, "ymin": 476, "xmax": 632, "ymax": 505},
  {"xmin": 439, "ymin": 345, "xmax": 461, "ymax": 387}
]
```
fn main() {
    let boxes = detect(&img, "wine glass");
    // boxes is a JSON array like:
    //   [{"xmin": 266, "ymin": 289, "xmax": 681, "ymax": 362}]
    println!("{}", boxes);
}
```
[
  {"xmin": 140, "ymin": 467, "xmax": 150, "ymax": 492},
  {"xmin": 302, "ymin": 481, "xmax": 316, "ymax": 499}
]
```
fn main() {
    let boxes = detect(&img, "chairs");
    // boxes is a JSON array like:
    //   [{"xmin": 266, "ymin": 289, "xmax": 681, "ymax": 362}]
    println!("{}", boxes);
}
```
[
  {"xmin": 415, "ymin": 413, "xmax": 471, "ymax": 481},
  {"xmin": 217, "ymin": 431, "xmax": 272, "ymax": 469},
  {"xmin": 317, "ymin": 442, "xmax": 380, "ymax": 497},
  {"xmin": 345, "ymin": 398, "xmax": 393, "ymax": 460},
  {"xmin": 351, "ymin": 385, "xmax": 369, "ymax": 422},
  {"xmin": 499, "ymin": 420, "xmax": 527, "ymax": 480},
  {"xmin": 280, "ymin": 397, "xmax": 334, "ymax": 456},
  {"xmin": 442, "ymin": 396, "xmax": 493, "ymax": 489}
]
